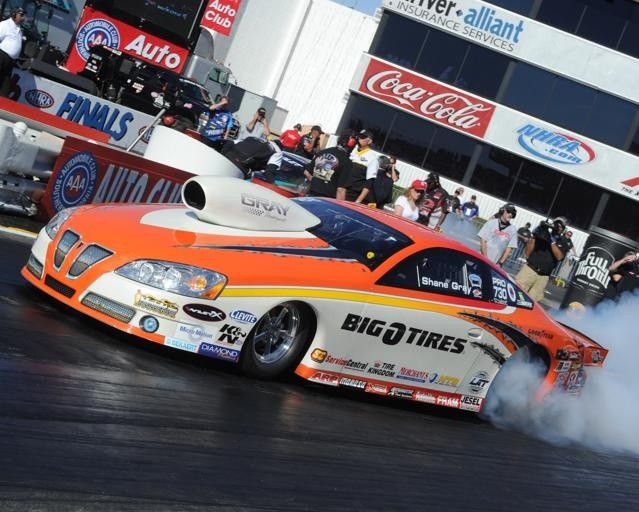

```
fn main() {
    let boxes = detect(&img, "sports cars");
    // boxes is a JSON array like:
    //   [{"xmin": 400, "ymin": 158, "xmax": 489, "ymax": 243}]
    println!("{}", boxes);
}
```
[{"xmin": 21, "ymin": 166, "xmax": 608, "ymax": 436}]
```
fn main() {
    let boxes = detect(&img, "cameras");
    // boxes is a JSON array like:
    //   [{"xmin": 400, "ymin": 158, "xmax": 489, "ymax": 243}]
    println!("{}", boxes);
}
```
[{"xmin": 259, "ymin": 112, "xmax": 265, "ymax": 116}]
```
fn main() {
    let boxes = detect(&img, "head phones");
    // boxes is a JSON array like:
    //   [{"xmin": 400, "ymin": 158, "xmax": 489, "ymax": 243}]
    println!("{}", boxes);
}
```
[
  {"xmin": 500, "ymin": 203, "xmax": 516, "ymax": 219},
  {"xmin": 13, "ymin": 8, "xmax": 23, "ymax": 18}
]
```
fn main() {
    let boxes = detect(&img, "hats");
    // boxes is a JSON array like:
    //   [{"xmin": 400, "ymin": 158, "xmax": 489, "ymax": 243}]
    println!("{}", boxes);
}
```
[
  {"xmin": 312, "ymin": 126, "xmax": 325, "ymax": 134},
  {"xmin": 358, "ymin": 129, "xmax": 374, "ymax": 139},
  {"xmin": 503, "ymin": 204, "xmax": 516, "ymax": 213},
  {"xmin": 413, "ymin": 180, "xmax": 428, "ymax": 190},
  {"xmin": 554, "ymin": 217, "xmax": 565, "ymax": 227},
  {"xmin": 258, "ymin": 107, "xmax": 265, "ymax": 116}
]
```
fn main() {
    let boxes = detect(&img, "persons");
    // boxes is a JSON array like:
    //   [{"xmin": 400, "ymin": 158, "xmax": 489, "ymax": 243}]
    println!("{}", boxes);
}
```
[{"xmin": 0, "ymin": 7, "xmax": 27, "ymax": 83}]
